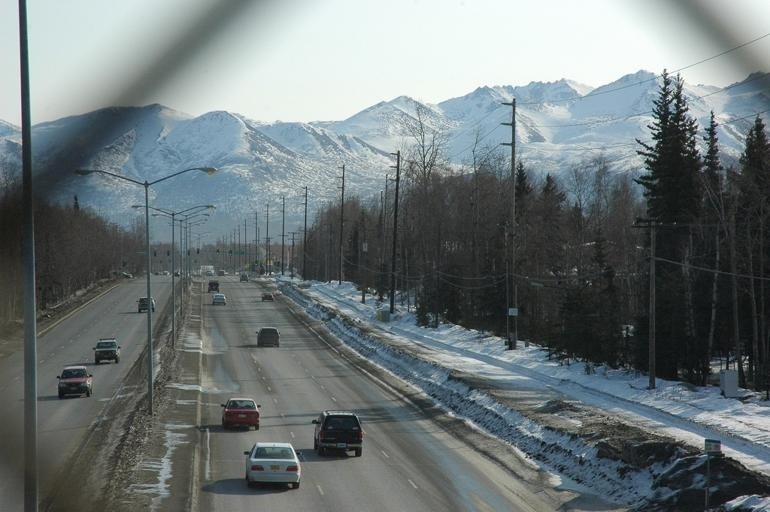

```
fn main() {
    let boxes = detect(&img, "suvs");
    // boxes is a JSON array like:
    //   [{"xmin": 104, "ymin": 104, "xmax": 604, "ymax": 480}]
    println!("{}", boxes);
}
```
[
  {"xmin": 311, "ymin": 411, "xmax": 363, "ymax": 457},
  {"xmin": 92, "ymin": 338, "xmax": 122, "ymax": 365}
]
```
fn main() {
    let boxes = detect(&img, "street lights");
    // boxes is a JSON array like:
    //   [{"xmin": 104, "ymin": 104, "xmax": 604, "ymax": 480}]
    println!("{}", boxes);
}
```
[
  {"xmin": 76, "ymin": 164, "xmax": 221, "ymax": 419},
  {"xmin": 131, "ymin": 204, "xmax": 217, "ymax": 350}
]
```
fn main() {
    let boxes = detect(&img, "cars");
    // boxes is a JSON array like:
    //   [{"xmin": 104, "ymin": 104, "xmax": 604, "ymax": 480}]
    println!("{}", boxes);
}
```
[
  {"xmin": 208, "ymin": 280, "xmax": 220, "ymax": 294},
  {"xmin": 244, "ymin": 441, "xmax": 300, "ymax": 489},
  {"xmin": 262, "ymin": 291, "xmax": 274, "ymax": 302},
  {"xmin": 256, "ymin": 327, "xmax": 280, "ymax": 347},
  {"xmin": 136, "ymin": 296, "xmax": 156, "ymax": 313},
  {"xmin": 212, "ymin": 293, "xmax": 227, "ymax": 306},
  {"xmin": 240, "ymin": 274, "xmax": 249, "ymax": 282},
  {"xmin": 220, "ymin": 397, "xmax": 261, "ymax": 430}
]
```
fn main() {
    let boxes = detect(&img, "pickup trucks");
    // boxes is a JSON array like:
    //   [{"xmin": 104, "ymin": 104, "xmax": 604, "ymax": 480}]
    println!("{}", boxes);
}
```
[{"xmin": 57, "ymin": 366, "xmax": 94, "ymax": 399}]
